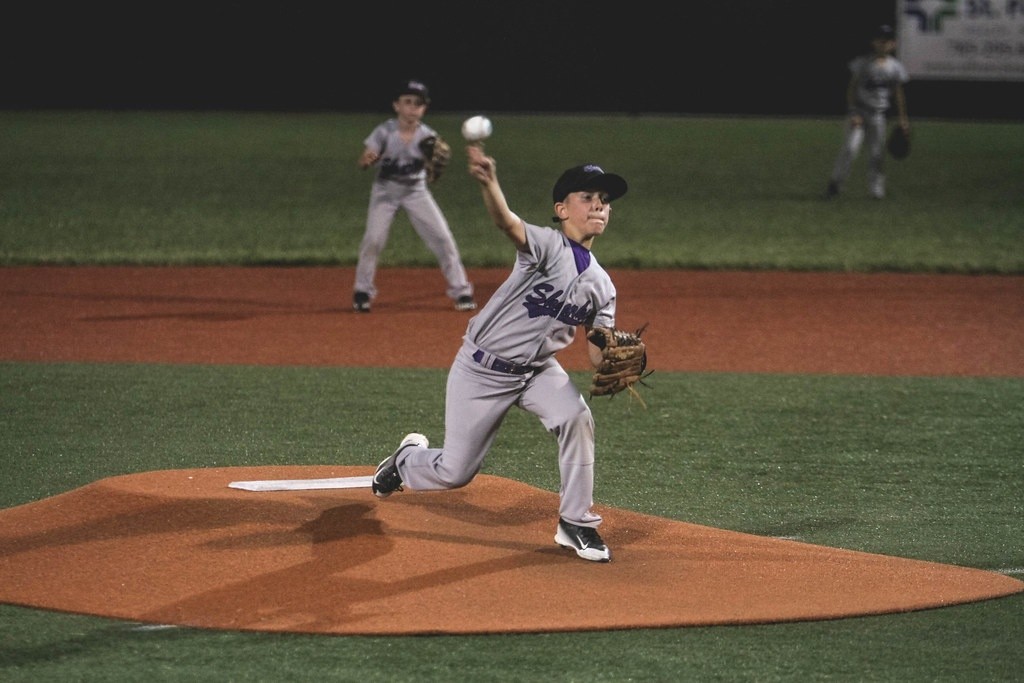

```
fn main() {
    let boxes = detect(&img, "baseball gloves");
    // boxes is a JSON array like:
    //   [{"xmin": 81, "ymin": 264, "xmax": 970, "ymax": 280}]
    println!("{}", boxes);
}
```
[
  {"xmin": 886, "ymin": 126, "xmax": 913, "ymax": 161},
  {"xmin": 584, "ymin": 321, "xmax": 657, "ymax": 409},
  {"xmin": 418, "ymin": 135, "xmax": 453, "ymax": 167}
]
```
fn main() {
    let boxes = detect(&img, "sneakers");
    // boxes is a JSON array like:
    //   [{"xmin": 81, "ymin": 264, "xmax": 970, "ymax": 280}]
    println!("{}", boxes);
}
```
[
  {"xmin": 371, "ymin": 433, "xmax": 430, "ymax": 498},
  {"xmin": 554, "ymin": 518, "xmax": 609, "ymax": 561}
]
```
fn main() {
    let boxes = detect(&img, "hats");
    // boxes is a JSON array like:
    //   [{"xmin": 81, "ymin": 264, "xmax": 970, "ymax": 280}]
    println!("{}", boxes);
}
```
[
  {"xmin": 395, "ymin": 81, "xmax": 429, "ymax": 102},
  {"xmin": 553, "ymin": 164, "xmax": 628, "ymax": 222},
  {"xmin": 875, "ymin": 23, "xmax": 896, "ymax": 42}
]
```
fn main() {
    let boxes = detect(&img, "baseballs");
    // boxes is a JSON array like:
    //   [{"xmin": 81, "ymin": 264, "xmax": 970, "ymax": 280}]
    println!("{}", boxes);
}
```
[{"xmin": 460, "ymin": 115, "xmax": 493, "ymax": 142}]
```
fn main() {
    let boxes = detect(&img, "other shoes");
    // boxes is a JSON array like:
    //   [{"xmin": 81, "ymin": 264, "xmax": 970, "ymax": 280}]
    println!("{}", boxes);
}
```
[
  {"xmin": 353, "ymin": 291, "xmax": 371, "ymax": 311},
  {"xmin": 455, "ymin": 295, "xmax": 478, "ymax": 310},
  {"xmin": 818, "ymin": 185, "xmax": 845, "ymax": 212},
  {"xmin": 866, "ymin": 175, "xmax": 886, "ymax": 199}
]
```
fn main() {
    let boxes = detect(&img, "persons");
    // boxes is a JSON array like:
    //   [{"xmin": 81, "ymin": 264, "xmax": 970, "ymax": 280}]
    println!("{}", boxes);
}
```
[
  {"xmin": 352, "ymin": 78, "xmax": 478, "ymax": 312},
  {"xmin": 371, "ymin": 140, "xmax": 648, "ymax": 564},
  {"xmin": 824, "ymin": 29, "xmax": 913, "ymax": 203}
]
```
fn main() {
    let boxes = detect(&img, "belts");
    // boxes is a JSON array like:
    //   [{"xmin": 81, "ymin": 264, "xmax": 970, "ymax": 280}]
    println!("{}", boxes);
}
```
[{"xmin": 473, "ymin": 350, "xmax": 533, "ymax": 374}]
